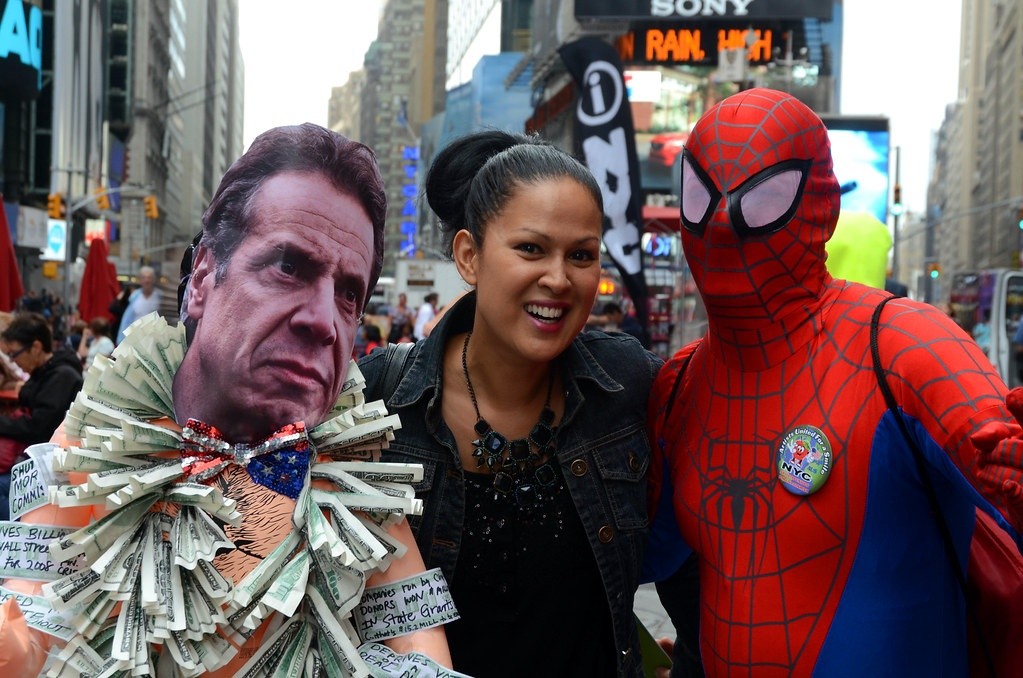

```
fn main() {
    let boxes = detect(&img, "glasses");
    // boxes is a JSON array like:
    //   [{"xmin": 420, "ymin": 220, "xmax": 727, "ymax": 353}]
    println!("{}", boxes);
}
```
[{"xmin": 7, "ymin": 339, "xmax": 38, "ymax": 361}]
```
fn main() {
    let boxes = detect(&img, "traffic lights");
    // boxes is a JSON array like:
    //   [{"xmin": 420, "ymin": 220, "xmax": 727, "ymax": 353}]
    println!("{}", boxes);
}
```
[
  {"xmin": 928, "ymin": 264, "xmax": 942, "ymax": 279},
  {"xmin": 892, "ymin": 185, "xmax": 903, "ymax": 217},
  {"xmin": 1017, "ymin": 201, "xmax": 1023, "ymax": 230},
  {"xmin": 95, "ymin": 187, "xmax": 109, "ymax": 211},
  {"xmin": 143, "ymin": 196, "xmax": 159, "ymax": 219},
  {"xmin": 47, "ymin": 194, "xmax": 60, "ymax": 220}
]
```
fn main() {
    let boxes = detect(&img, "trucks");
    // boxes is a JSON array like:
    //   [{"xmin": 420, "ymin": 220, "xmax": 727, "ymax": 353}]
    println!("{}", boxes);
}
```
[{"xmin": 366, "ymin": 276, "xmax": 396, "ymax": 311}]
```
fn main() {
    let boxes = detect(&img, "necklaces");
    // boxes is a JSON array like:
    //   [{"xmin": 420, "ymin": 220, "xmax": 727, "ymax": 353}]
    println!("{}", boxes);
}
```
[{"xmin": 462, "ymin": 330, "xmax": 557, "ymax": 505}]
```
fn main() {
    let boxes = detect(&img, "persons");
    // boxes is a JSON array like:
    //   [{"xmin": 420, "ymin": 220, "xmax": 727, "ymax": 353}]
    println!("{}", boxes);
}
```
[
  {"xmin": 647, "ymin": 88, "xmax": 1023, "ymax": 678},
  {"xmin": 603, "ymin": 302, "xmax": 652, "ymax": 351},
  {"xmin": 78, "ymin": 266, "xmax": 162, "ymax": 376},
  {"xmin": 344, "ymin": 128, "xmax": 700, "ymax": 678},
  {"xmin": 0, "ymin": 315, "xmax": 83, "ymax": 443},
  {"xmin": 0, "ymin": 124, "xmax": 454, "ymax": 678}
]
